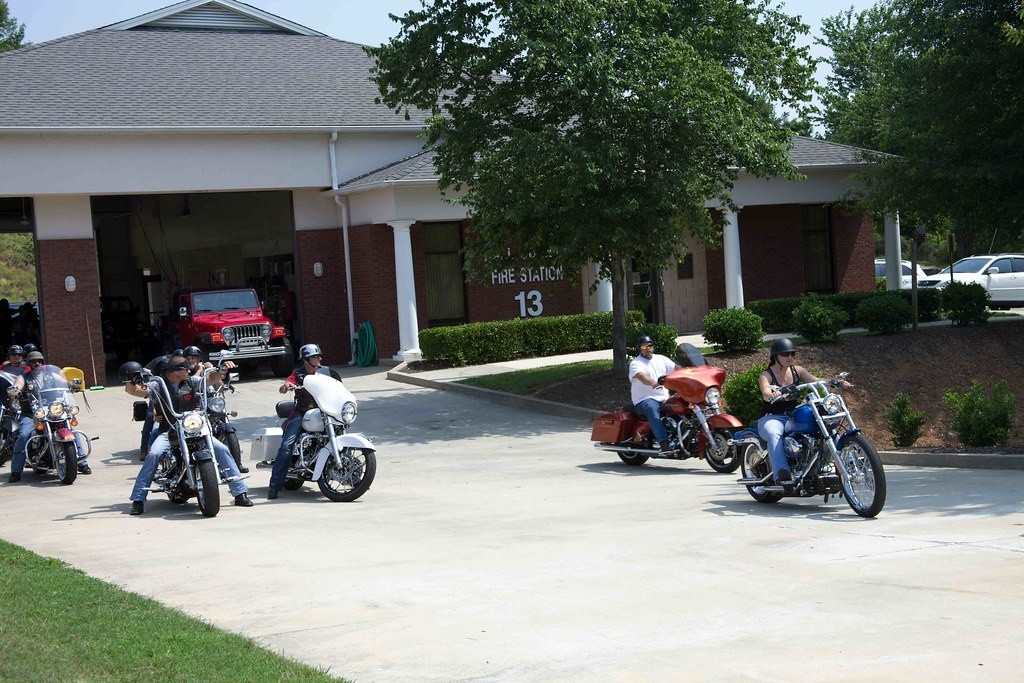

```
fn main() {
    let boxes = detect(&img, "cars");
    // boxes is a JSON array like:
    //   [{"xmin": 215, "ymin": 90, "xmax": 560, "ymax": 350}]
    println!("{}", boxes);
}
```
[{"xmin": 874, "ymin": 258, "xmax": 928, "ymax": 290}]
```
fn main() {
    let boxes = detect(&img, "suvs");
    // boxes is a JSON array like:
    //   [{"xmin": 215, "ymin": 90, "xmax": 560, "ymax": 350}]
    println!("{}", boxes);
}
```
[
  {"xmin": 917, "ymin": 251, "xmax": 1024, "ymax": 310},
  {"xmin": 163, "ymin": 288, "xmax": 294, "ymax": 382}
]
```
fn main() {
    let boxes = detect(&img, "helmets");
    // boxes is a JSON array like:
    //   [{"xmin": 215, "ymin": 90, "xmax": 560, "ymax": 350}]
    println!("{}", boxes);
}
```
[
  {"xmin": 26, "ymin": 351, "xmax": 46, "ymax": 366},
  {"xmin": 8, "ymin": 345, "xmax": 23, "ymax": 358},
  {"xmin": 298, "ymin": 344, "xmax": 323, "ymax": 360},
  {"xmin": 173, "ymin": 349, "xmax": 184, "ymax": 357},
  {"xmin": 638, "ymin": 335, "xmax": 653, "ymax": 352},
  {"xmin": 770, "ymin": 338, "xmax": 796, "ymax": 356},
  {"xmin": 119, "ymin": 362, "xmax": 143, "ymax": 381},
  {"xmin": 183, "ymin": 346, "xmax": 201, "ymax": 358},
  {"xmin": 23, "ymin": 344, "xmax": 38, "ymax": 356}
]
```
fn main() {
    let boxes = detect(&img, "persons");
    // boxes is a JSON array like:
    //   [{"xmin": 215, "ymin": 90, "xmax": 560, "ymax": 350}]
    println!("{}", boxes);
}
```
[
  {"xmin": 628, "ymin": 336, "xmax": 684, "ymax": 452},
  {"xmin": 0, "ymin": 298, "xmax": 41, "ymax": 366},
  {"xmin": 266, "ymin": 343, "xmax": 323, "ymax": 500},
  {"xmin": 124, "ymin": 347, "xmax": 254, "ymax": 515},
  {"xmin": 758, "ymin": 337, "xmax": 854, "ymax": 493},
  {"xmin": 0, "ymin": 344, "xmax": 92, "ymax": 483}
]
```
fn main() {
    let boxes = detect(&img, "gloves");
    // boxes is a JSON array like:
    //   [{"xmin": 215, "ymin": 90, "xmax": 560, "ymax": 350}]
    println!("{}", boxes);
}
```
[{"xmin": 658, "ymin": 376, "xmax": 666, "ymax": 385}]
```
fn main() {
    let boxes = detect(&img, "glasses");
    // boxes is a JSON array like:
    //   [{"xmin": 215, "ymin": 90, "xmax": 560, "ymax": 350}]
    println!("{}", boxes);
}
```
[
  {"xmin": 640, "ymin": 343, "xmax": 654, "ymax": 350},
  {"xmin": 779, "ymin": 351, "xmax": 795, "ymax": 357},
  {"xmin": 27, "ymin": 359, "xmax": 43, "ymax": 364},
  {"xmin": 307, "ymin": 354, "xmax": 322, "ymax": 359},
  {"xmin": 10, "ymin": 353, "xmax": 22, "ymax": 358}
]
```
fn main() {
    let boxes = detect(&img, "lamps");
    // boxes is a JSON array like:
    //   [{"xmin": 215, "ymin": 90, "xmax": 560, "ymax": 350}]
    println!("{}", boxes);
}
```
[
  {"xmin": 314, "ymin": 261, "xmax": 324, "ymax": 277},
  {"xmin": 65, "ymin": 276, "xmax": 76, "ymax": 292}
]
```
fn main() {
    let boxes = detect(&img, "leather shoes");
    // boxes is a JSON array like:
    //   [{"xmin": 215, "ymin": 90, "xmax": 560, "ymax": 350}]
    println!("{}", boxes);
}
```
[
  {"xmin": 234, "ymin": 493, "xmax": 253, "ymax": 507},
  {"xmin": 131, "ymin": 499, "xmax": 144, "ymax": 514}
]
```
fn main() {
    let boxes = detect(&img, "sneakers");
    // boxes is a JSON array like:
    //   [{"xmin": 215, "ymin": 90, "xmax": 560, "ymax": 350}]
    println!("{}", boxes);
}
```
[
  {"xmin": 268, "ymin": 486, "xmax": 279, "ymax": 499},
  {"xmin": 8, "ymin": 471, "xmax": 21, "ymax": 483},
  {"xmin": 78, "ymin": 465, "xmax": 91, "ymax": 474}
]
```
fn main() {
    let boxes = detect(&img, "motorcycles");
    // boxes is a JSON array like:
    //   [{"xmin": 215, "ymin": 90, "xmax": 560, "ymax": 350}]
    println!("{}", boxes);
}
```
[
  {"xmin": 726, "ymin": 372, "xmax": 887, "ymax": 519},
  {"xmin": 249, "ymin": 366, "xmax": 377, "ymax": 503},
  {"xmin": 120, "ymin": 349, "xmax": 246, "ymax": 519},
  {"xmin": 0, "ymin": 363, "xmax": 100, "ymax": 484},
  {"xmin": 589, "ymin": 342, "xmax": 746, "ymax": 474}
]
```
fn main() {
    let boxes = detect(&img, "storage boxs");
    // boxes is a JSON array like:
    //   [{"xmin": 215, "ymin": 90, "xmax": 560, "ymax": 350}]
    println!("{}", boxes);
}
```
[
  {"xmin": 591, "ymin": 411, "xmax": 634, "ymax": 443},
  {"xmin": 249, "ymin": 427, "xmax": 284, "ymax": 460},
  {"xmin": 133, "ymin": 401, "xmax": 148, "ymax": 421}
]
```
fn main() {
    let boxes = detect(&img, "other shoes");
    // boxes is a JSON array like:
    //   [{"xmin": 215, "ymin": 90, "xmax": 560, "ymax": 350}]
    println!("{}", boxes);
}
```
[
  {"xmin": 777, "ymin": 468, "xmax": 793, "ymax": 494},
  {"xmin": 238, "ymin": 464, "xmax": 249, "ymax": 472},
  {"xmin": 140, "ymin": 451, "xmax": 148, "ymax": 461},
  {"xmin": 659, "ymin": 440, "xmax": 671, "ymax": 451}
]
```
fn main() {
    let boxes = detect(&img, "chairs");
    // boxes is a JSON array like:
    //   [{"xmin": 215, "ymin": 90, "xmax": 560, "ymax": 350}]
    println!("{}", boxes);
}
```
[{"xmin": 60, "ymin": 367, "xmax": 91, "ymax": 412}]
